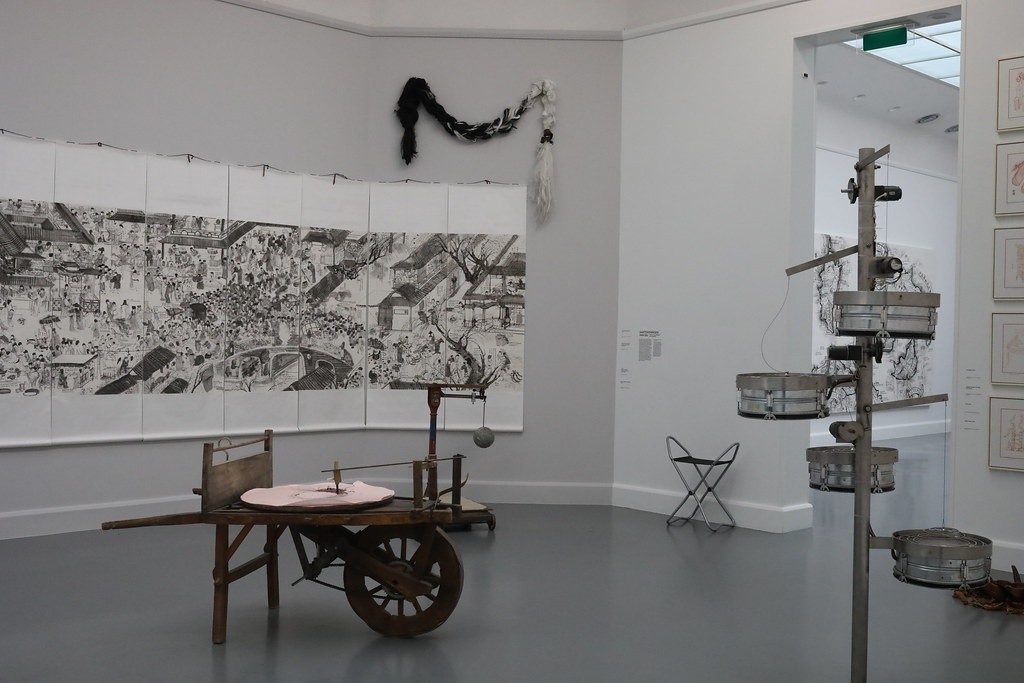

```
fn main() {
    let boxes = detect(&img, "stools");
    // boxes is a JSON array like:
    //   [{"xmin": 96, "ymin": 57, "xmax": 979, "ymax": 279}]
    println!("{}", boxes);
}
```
[{"xmin": 665, "ymin": 436, "xmax": 740, "ymax": 532}]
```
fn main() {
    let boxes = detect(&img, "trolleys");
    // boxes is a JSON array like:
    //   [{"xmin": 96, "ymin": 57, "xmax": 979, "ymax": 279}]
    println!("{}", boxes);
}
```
[{"xmin": 101, "ymin": 427, "xmax": 465, "ymax": 644}]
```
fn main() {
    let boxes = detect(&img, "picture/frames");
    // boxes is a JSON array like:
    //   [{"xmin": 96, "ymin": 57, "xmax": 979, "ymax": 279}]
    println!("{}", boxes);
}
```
[
  {"xmin": 992, "ymin": 227, "xmax": 1024, "ymax": 301},
  {"xmin": 995, "ymin": 141, "xmax": 1024, "ymax": 217},
  {"xmin": 988, "ymin": 395, "xmax": 1024, "ymax": 473},
  {"xmin": 990, "ymin": 312, "xmax": 1024, "ymax": 386},
  {"xmin": 996, "ymin": 56, "xmax": 1024, "ymax": 132}
]
